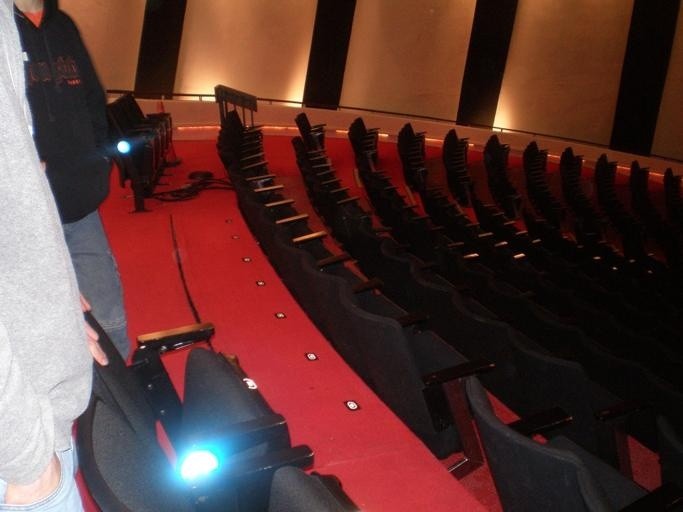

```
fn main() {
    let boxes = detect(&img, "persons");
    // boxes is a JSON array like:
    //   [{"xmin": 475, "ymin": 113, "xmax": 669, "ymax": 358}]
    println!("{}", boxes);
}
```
[{"xmin": 0, "ymin": 2, "xmax": 129, "ymax": 512}]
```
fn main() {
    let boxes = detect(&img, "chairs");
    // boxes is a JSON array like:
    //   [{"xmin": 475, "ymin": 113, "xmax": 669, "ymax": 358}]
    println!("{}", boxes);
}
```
[
  {"xmin": 76, "ymin": 313, "xmax": 359, "ymax": 512},
  {"xmin": 215, "ymin": 109, "xmax": 683, "ymax": 512},
  {"xmin": 106, "ymin": 90, "xmax": 182, "ymax": 215}
]
